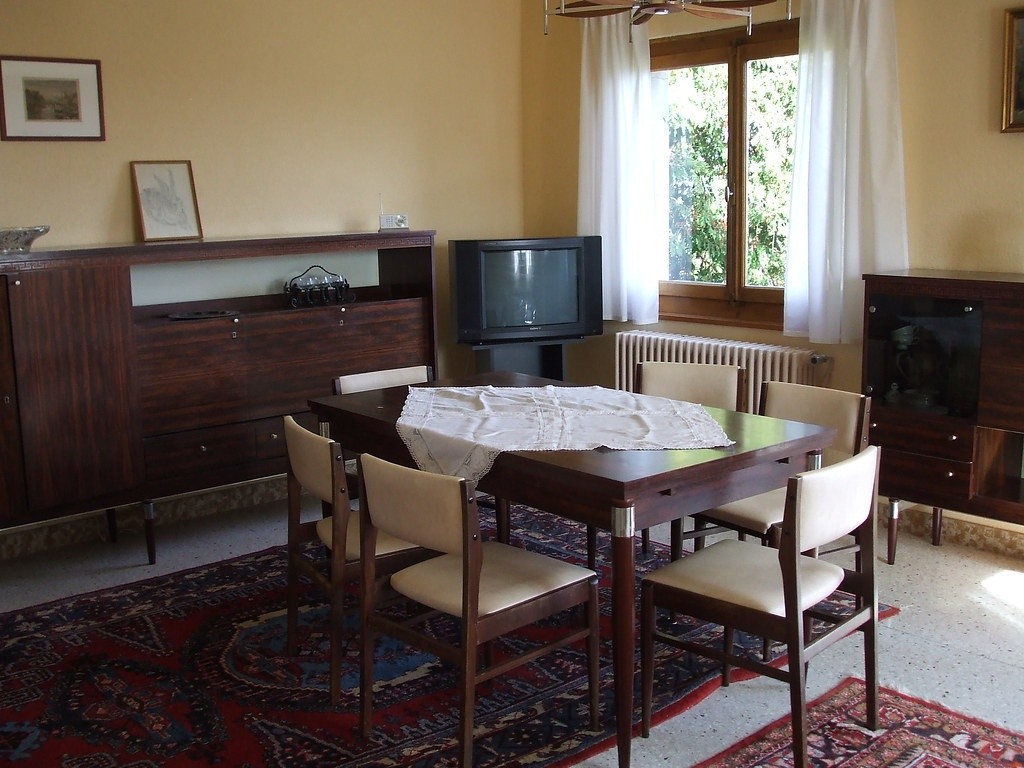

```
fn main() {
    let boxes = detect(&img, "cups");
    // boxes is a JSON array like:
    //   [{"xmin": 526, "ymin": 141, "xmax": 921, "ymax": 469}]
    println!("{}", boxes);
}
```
[{"xmin": 892, "ymin": 323, "xmax": 921, "ymax": 350}]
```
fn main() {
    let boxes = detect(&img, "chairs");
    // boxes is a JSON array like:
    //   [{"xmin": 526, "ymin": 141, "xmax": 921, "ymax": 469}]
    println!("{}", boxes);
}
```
[{"xmin": 282, "ymin": 360, "xmax": 880, "ymax": 768}]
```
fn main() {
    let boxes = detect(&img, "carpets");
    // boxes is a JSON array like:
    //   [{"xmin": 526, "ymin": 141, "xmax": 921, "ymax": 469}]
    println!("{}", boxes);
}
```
[
  {"xmin": 0, "ymin": 494, "xmax": 903, "ymax": 768},
  {"xmin": 694, "ymin": 673, "xmax": 1024, "ymax": 768}
]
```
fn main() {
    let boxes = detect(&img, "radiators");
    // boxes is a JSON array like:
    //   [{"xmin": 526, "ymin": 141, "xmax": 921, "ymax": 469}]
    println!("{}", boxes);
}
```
[{"xmin": 614, "ymin": 329, "xmax": 818, "ymax": 415}]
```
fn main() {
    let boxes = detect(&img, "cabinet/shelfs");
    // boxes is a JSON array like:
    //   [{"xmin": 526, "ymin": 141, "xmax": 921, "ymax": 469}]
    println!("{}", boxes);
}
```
[
  {"xmin": 856, "ymin": 267, "xmax": 1024, "ymax": 565},
  {"xmin": 0, "ymin": 231, "xmax": 441, "ymax": 566}
]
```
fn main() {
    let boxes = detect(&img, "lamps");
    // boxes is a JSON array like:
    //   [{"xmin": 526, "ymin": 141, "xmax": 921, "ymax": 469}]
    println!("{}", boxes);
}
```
[{"xmin": 544, "ymin": 0, "xmax": 791, "ymax": 44}]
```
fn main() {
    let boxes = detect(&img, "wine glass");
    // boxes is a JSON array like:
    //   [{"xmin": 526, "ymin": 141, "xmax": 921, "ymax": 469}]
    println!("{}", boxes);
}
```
[
  {"xmin": 312, "ymin": 276, "xmax": 331, "ymax": 303},
  {"xmin": 299, "ymin": 276, "xmax": 314, "ymax": 304},
  {"xmin": 330, "ymin": 275, "xmax": 344, "ymax": 301}
]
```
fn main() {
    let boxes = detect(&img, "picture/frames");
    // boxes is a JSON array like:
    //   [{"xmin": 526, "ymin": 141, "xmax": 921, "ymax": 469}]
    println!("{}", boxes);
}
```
[
  {"xmin": 129, "ymin": 160, "xmax": 203, "ymax": 242},
  {"xmin": 0, "ymin": 55, "xmax": 107, "ymax": 141},
  {"xmin": 999, "ymin": 8, "xmax": 1024, "ymax": 133}
]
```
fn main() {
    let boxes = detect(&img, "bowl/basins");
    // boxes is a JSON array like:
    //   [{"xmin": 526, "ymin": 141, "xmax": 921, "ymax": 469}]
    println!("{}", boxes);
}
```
[{"xmin": 0, "ymin": 226, "xmax": 50, "ymax": 255}]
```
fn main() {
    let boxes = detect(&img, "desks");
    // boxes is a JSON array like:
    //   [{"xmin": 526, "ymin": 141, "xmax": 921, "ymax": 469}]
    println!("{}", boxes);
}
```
[
  {"xmin": 473, "ymin": 338, "xmax": 589, "ymax": 382},
  {"xmin": 307, "ymin": 370, "xmax": 838, "ymax": 767}
]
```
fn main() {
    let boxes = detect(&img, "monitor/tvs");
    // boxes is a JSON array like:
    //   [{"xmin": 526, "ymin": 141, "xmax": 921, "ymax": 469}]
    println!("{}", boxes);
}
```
[{"xmin": 455, "ymin": 236, "xmax": 604, "ymax": 346}]
